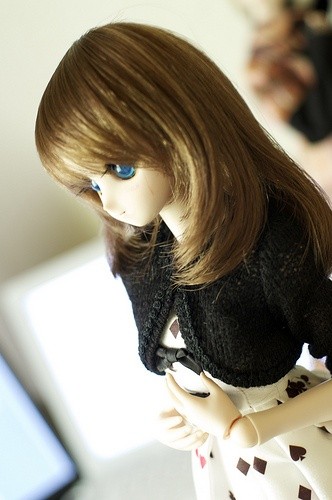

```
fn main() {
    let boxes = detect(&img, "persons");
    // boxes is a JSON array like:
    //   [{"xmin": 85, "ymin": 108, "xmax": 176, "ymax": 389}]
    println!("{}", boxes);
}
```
[{"xmin": 33, "ymin": 20, "xmax": 332, "ymax": 499}]
